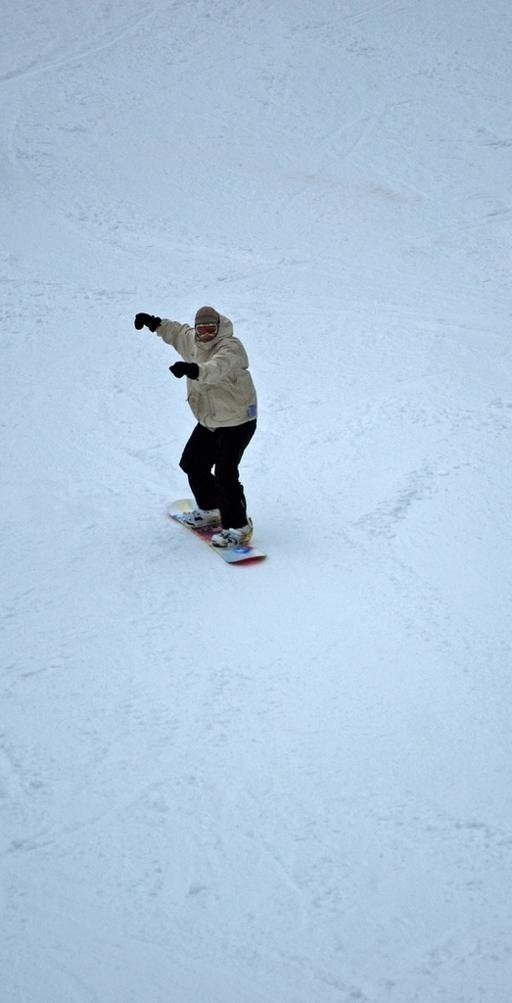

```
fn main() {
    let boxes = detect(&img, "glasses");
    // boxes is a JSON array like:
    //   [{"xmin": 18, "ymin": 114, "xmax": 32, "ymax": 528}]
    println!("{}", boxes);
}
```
[{"xmin": 195, "ymin": 323, "xmax": 218, "ymax": 337}]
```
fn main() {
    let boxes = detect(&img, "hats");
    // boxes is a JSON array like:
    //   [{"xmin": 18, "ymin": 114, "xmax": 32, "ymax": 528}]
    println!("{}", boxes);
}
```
[{"xmin": 194, "ymin": 305, "xmax": 219, "ymax": 323}]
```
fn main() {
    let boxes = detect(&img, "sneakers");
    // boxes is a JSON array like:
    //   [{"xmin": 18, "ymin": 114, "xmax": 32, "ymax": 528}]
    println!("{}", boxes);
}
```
[
  {"xmin": 211, "ymin": 525, "xmax": 253, "ymax": 547},
  {"xmin": 177, "ymin": 507, "xmax": 222, "ymax": 529}
]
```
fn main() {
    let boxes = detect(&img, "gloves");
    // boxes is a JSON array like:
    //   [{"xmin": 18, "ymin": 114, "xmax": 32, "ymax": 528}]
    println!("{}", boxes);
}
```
[
  {"xmin": 168, "ymin": 361, "xmax": 200, "ymax": 380},
  {"xmin": 134, "ymin": 312, "xmax": 161, "ymax": 332}
]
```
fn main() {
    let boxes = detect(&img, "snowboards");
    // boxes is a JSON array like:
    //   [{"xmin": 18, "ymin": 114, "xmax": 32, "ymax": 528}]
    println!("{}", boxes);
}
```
[{"xmin": 170, "ymin": 499, "xmax": 267, "ymax": 563}]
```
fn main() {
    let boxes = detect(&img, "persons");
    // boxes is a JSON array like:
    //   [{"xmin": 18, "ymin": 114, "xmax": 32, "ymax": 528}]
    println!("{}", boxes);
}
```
[{"xmin": 133, "ymin": 302, "xmax": 260, "ymax": 550}]
